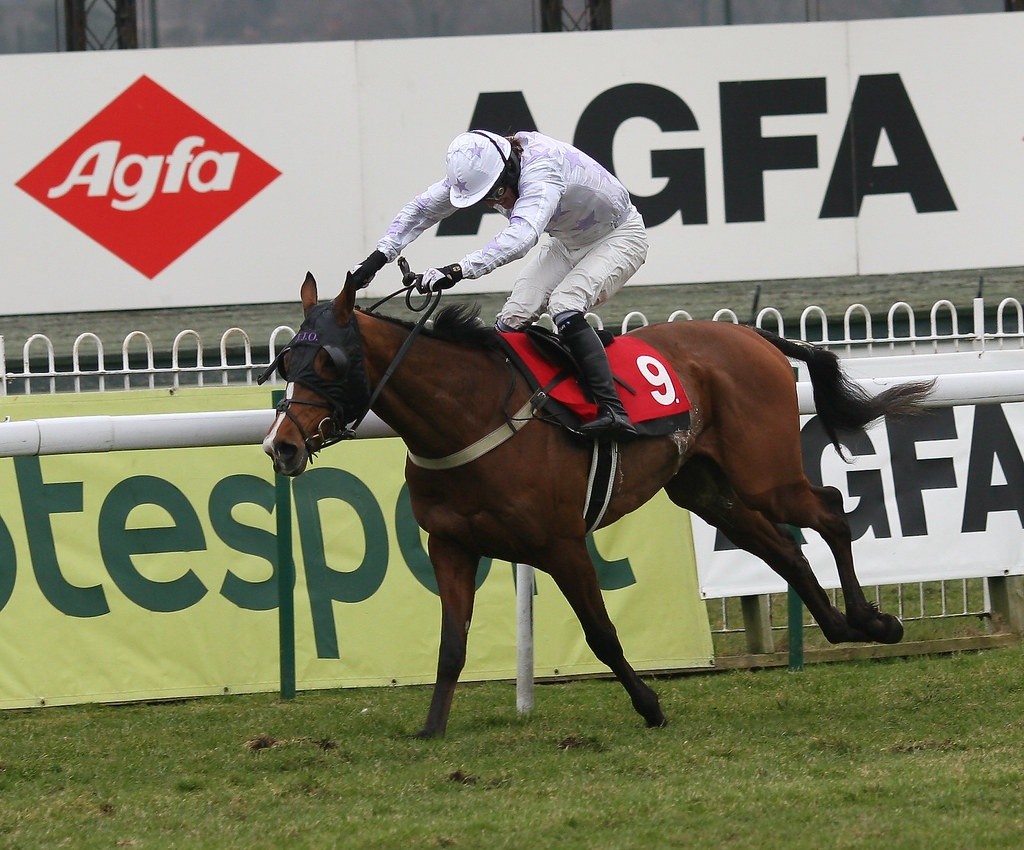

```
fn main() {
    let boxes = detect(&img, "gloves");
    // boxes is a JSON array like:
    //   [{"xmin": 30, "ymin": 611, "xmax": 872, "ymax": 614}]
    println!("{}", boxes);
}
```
[
  {"xmin": 351, "ymin": 249, "xmax": 388, "ymax": 288},
  {"xmin": 421, "ymin": 263, "xmax": 463, "ymax": 292}
]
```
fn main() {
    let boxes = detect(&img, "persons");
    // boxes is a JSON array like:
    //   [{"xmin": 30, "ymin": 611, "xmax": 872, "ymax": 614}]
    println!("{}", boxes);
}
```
[{"xmin": 352, "ymin": 130, "xmax": 651, "ymax": 439}]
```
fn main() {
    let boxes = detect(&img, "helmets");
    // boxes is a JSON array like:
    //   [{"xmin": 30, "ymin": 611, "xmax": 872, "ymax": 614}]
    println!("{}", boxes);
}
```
[{"xmin": 446, "ymin": 129, "xmax": 511, "ymax": 208}]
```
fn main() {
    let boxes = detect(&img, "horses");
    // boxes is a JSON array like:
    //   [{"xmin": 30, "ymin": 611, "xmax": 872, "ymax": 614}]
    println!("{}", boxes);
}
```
[{"xmin": 262, "ymin": 271, "xmax": 942, "ymax": 740}]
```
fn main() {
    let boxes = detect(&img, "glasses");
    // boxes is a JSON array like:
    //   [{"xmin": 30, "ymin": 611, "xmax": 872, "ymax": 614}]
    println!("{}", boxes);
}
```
[{"xmin": 483, "ymin": 178, "xmax": 508, "ymax": 205}]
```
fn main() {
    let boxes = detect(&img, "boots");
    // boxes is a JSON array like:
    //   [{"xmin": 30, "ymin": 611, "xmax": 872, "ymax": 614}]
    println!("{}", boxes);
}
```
[{"xmin": 564, "ymin": 327, "xmax": 637, "ymax": 437}]
